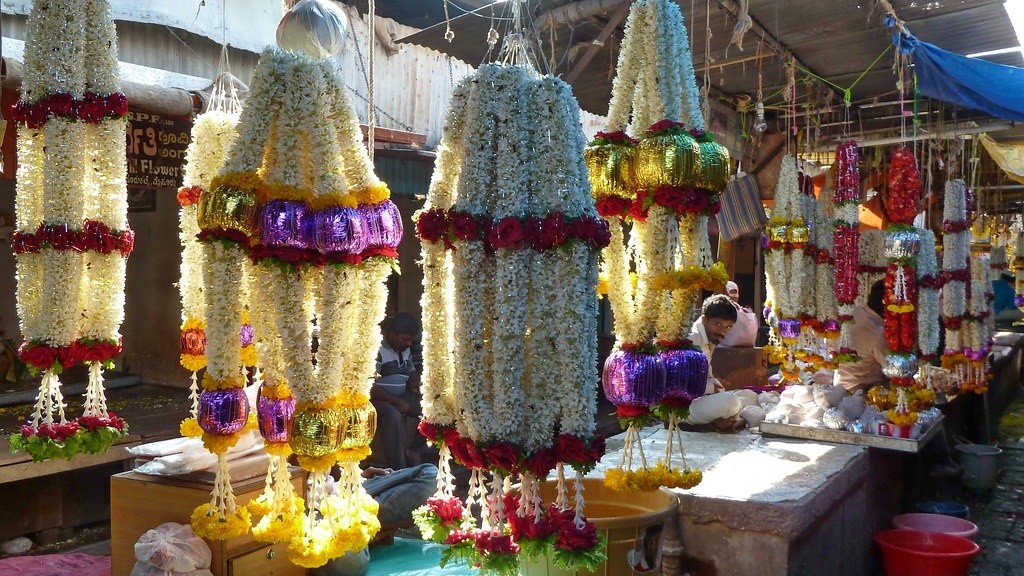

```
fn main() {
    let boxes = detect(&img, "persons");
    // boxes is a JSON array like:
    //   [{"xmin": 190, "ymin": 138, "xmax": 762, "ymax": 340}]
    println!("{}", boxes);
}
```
[
  {"xmin": 838, "ymin": 277, "xmax": 891, "ymax": 396},
  {"xmin": 300, "ymin": 462, "xmax": 440, "ymax": 545},
  {"xmin": 668, "ymin": 280, "xmax": 758, "ymax": 432},
  {"xmin": 359, "ymin": 312, "xmax": 428, "ymax": 470},
  {"xmin": 992, "ymin": 269, "xmax": 1024, "ymax": 321}
]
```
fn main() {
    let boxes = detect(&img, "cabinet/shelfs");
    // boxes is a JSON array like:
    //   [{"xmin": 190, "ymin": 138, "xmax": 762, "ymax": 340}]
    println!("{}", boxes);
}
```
[{"xmin": 110, "ymin": 465, "xmax": 310, "ymax": 576}]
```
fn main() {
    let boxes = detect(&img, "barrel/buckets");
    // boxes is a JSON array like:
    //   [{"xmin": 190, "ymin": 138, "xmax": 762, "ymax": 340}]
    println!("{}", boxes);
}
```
[
  {"xmin": 874, "ymin": 527, "xmax": 980, "ymax": 576},
  {"xmin": 916, "ymin": 501, "xmax": 971, "ymax": 520},
  {"xmin": 506, "ymin": 475, "xmax": 679, "ymax": 576},
  {"xmin": 891, "ymin": 512, "xmax": 979, "ymax": 540},
  {"xmin": 951, "ymin": 433, "xmax": 1003, "ymax": 490}
]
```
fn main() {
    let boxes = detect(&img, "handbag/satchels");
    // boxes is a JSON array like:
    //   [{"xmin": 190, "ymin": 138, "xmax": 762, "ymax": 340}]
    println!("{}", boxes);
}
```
[{"xmin": 134, "ymin": 520, "xmax": 212, "ymax": 572}]
